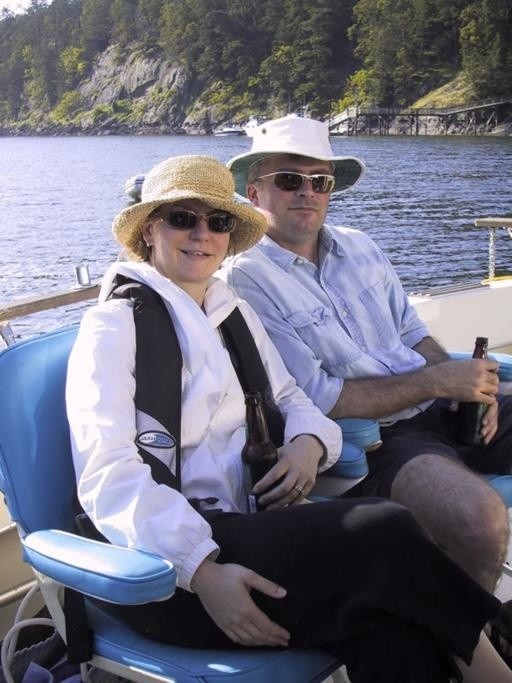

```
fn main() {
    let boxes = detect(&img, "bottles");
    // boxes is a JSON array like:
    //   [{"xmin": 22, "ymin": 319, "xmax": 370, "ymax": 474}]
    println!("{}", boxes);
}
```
[
  {"xmin": 454, "ymin": 335, "xmax": 491, "ymax": 446},
  {"xmin": 241, "ymin": 390, "xmax": 288, "ymax": 515}
]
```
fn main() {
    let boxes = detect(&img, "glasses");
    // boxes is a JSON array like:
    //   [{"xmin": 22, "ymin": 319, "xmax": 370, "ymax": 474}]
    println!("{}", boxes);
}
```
[
  {"xmin": 257, "ymin": 170, "xmax": 337, "ymax": 193},
  {"xmin": 149, "ymin": 208, "xmax": 237, "ymax": 232}
]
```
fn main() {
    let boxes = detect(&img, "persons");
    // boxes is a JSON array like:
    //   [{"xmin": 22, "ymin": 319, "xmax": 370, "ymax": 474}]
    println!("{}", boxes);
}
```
[
  {"xmin": 213, "ymin": 114, "xmax": 511, "ymax": 595},
  {"xmin": 65, "ymin": 153, "xmax": 511, "ymax": 683}
]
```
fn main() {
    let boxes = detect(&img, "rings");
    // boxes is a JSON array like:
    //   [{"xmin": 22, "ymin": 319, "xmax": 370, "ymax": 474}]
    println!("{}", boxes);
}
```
[{"xmin": 294, "ymin": 482, "xmax": 304, "ymax": 494}]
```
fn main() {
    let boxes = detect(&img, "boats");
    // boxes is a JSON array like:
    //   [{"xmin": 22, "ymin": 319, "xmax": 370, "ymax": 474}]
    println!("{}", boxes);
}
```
[
  {"xmin": 1, "ymin": 216, "xmax": 512, "ymax": 680},
  {"xmin": 213, "ymin": 124, "xmax": 243, "ymax": 137}
]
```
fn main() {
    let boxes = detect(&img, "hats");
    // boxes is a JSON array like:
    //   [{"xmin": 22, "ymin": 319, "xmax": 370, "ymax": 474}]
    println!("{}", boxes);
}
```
[
  {"xmin": 109, "ymin": 152, "xmax": 270, "ymax": 263},
  {"xmin": 224, "ymin": 114, "xmax": 367, "ymax": 198}
]
